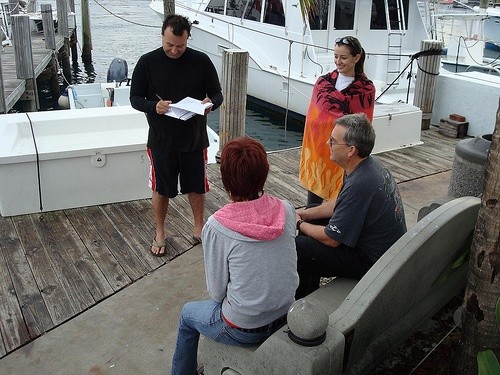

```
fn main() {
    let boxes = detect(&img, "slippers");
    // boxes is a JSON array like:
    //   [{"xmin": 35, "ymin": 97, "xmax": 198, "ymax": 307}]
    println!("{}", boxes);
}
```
[
  {"xmin": 151, "ymin": 235, "xmax": 166, "ymax": 256},
  {"xmin": 193, "ymin": 235, "xmax": 201, "ymax": 242}
]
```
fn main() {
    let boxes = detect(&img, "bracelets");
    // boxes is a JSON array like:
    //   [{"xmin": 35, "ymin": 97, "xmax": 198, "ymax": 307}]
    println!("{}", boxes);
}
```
[{"xmin": 296, "ymin": 219, "xmax": 303, "ymax": 231}]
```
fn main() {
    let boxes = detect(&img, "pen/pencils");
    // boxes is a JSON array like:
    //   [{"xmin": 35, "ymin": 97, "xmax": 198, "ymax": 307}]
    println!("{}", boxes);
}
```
[{"xmin": 155, "ymin": 93, "xmax": 171, "ymax": 109}]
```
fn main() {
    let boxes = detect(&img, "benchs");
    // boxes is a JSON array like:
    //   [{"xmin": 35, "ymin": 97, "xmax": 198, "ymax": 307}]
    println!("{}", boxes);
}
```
[{"xmin": 217, "ymin": 195, "xmax": 482, "ymax": 375}]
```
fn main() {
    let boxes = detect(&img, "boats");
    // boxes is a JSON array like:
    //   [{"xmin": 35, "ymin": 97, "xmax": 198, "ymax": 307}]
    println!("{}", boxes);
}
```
[
  {"xmin": 432, "ymin": 4, "xmax": 500, "ymax": 77},
  {"xmin": 149, "ymin": 0, "xmax": 441, "ymax": 128}
]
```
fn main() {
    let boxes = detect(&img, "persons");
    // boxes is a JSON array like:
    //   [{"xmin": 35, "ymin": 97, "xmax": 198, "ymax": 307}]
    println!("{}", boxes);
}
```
[
  {"xmin": 129, "ymin": 13, "xmax": 224, "ymax": 257},
  {"xmin": 300, "ymin": 36, "xmax": 376, "ymax": 210},
  {"xmin": 295, "ymin": 114, "xmax": 406, "ymax": 300},
  {"xmin": 171, "ymin": 136, "xmax": 300, "ymax": 375}
]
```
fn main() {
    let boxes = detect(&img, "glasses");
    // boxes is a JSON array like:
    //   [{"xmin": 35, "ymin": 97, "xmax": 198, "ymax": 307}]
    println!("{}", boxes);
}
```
[
  {"xmin": 329, "ymin": 137, "xmax": 349, "ymax": 147},
  {"xmin": 336, "ymin": 37, "xmax": 349, "ymax": 45}
]
